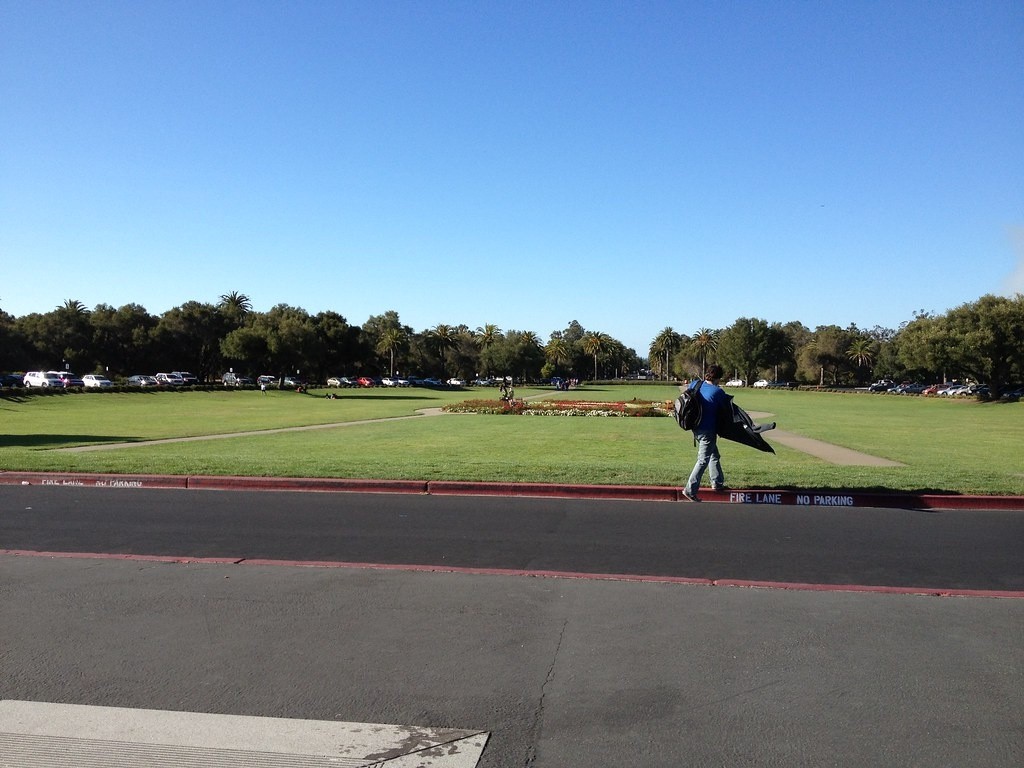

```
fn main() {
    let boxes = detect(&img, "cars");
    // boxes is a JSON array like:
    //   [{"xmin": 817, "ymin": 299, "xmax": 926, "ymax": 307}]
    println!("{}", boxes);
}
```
[
  {"xmin": 341, "ymin": 376, "xmax": 583, "ymax": 388},
  {"xmin": 81, "ymin": 374, "xmax": 111, "ymax": 388},
  {"xmin": 237, "ymin": 375, "xmax": 302, "ymax": 389},
  {"xmin": 0, "ymin": 375, "xmax": 24, "ymax": 388},
  {"xmin": 753, "ymin": 378, "xmax": 771, "ymax": 389},
  {"xmin": 600, "ymin": 368, "xmax": 659, "ymax": 382},
  {"xmin": 768, "ymin": 377, "xmax": 800, "ymax": 391},
  {"xmin": 127, "ymin": 374, "xmax": 158, "ymax": 387},
  {"xmin": 726, "ymin": 378, "xmax": 745, "ymax": 389},
  {"xmin": 327, "ymin": 377, "xmax": 348, "ymax": 388},
  {"xmin": 867, "ymin": 379, "xmax": 1024, "ymax": 402}
]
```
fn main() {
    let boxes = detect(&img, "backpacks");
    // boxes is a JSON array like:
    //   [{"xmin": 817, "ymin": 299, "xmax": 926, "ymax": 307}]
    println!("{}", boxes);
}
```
[{"xmin": 673, "ymin": 380, "xmax": 705, "ymax": 431}]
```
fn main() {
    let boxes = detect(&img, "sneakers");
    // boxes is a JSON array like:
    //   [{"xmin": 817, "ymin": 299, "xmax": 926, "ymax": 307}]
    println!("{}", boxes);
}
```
[
  {"xmin": 711, "ymin": 483, "xmax": 728, "ymax": 490},
  {"xmin": 682, "ymin": 489, "xmax": 702, "ymax": 503}
]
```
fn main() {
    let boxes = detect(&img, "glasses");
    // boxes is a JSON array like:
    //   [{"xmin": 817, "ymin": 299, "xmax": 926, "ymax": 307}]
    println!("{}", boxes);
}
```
[{"xmin": 719, "ymin": 377, "xmax": 723, "ymax": 381}]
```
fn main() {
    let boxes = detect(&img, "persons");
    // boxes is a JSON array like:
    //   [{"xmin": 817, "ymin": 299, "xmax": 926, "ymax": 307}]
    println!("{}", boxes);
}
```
[
  {"xmin": 296, "ymin": 386, "xmax": 308, "ymax": 394},
  {"xmin": 324, "ymin": 393, "xmax": 337, "ymax": 399},
  {"xmin": 260, "ymin": 383, "xmax": 267, "ymax": 395},
  {"xmin": 682, "ymin": 365, "xmax": 777, "ymax": 503}
]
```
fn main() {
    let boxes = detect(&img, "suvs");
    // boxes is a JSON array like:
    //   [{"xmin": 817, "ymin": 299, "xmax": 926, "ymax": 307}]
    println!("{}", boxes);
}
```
[
  {"xmin": 59, "ymin": 371, "xmax": 84, "ymax": 389},
  {"xmin": 171, "ymin": 371, "xmax": 200, "ymax": 386},
  {"xmin": 23, "ymin": 371, "xmax": 64, "ymax": 389},
  {"xmin": 155, "ymin": 373, "xmax": 185, "ymax": 386}
]
